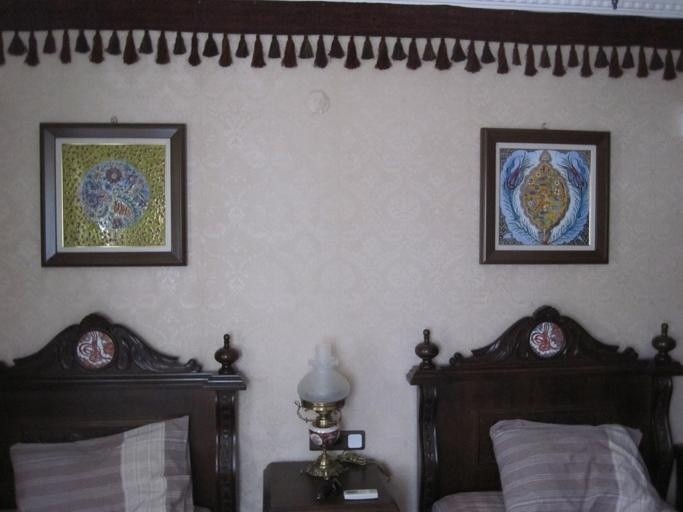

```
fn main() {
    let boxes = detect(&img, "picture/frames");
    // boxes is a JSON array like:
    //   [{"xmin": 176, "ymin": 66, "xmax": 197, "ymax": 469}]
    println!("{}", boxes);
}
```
[
  {"xmin": 480, "ymin": 129, "xmax": 610, "ymax": 265},
  {"xmin": 40, "ymin": 123, "xmax": 186, "ymax": 266}
]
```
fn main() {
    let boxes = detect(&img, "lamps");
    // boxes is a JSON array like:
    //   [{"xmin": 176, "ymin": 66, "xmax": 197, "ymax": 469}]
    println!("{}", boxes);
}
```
[{"xmin": 295, "ymin": 340, "xmax": 350, "ymax": 503}]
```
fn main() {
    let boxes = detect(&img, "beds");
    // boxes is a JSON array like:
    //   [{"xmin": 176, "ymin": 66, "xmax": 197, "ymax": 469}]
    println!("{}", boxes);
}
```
[
  {"xmin": 406, "ymin": 305, "xmax": 683, "ymax": 511},
  {"xmin": 2, "ymin": 314, "xmax": 248, "ymax": 511}
]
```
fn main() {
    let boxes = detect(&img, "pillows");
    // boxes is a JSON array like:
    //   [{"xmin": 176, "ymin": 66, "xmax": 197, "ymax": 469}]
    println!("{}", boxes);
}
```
[
  {"xmin": 9, "ymin": 413, "xmax": 196, "ymax": 512},
  {"xmin": 487, "ymin": 419, "xmax": 672, "ymax": 510}
]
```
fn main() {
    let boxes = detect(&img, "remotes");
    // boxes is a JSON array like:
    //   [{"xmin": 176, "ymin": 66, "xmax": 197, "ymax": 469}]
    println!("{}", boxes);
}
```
[{"xmin": 343, "ymin": 488, "xmax": 379, "ymax": 500}]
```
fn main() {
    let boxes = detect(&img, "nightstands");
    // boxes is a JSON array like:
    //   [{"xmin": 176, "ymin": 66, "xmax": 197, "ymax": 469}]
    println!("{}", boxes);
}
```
[{"xmin": 263, "ymin": 461, "xmax": 400, "ymax": 511}]
